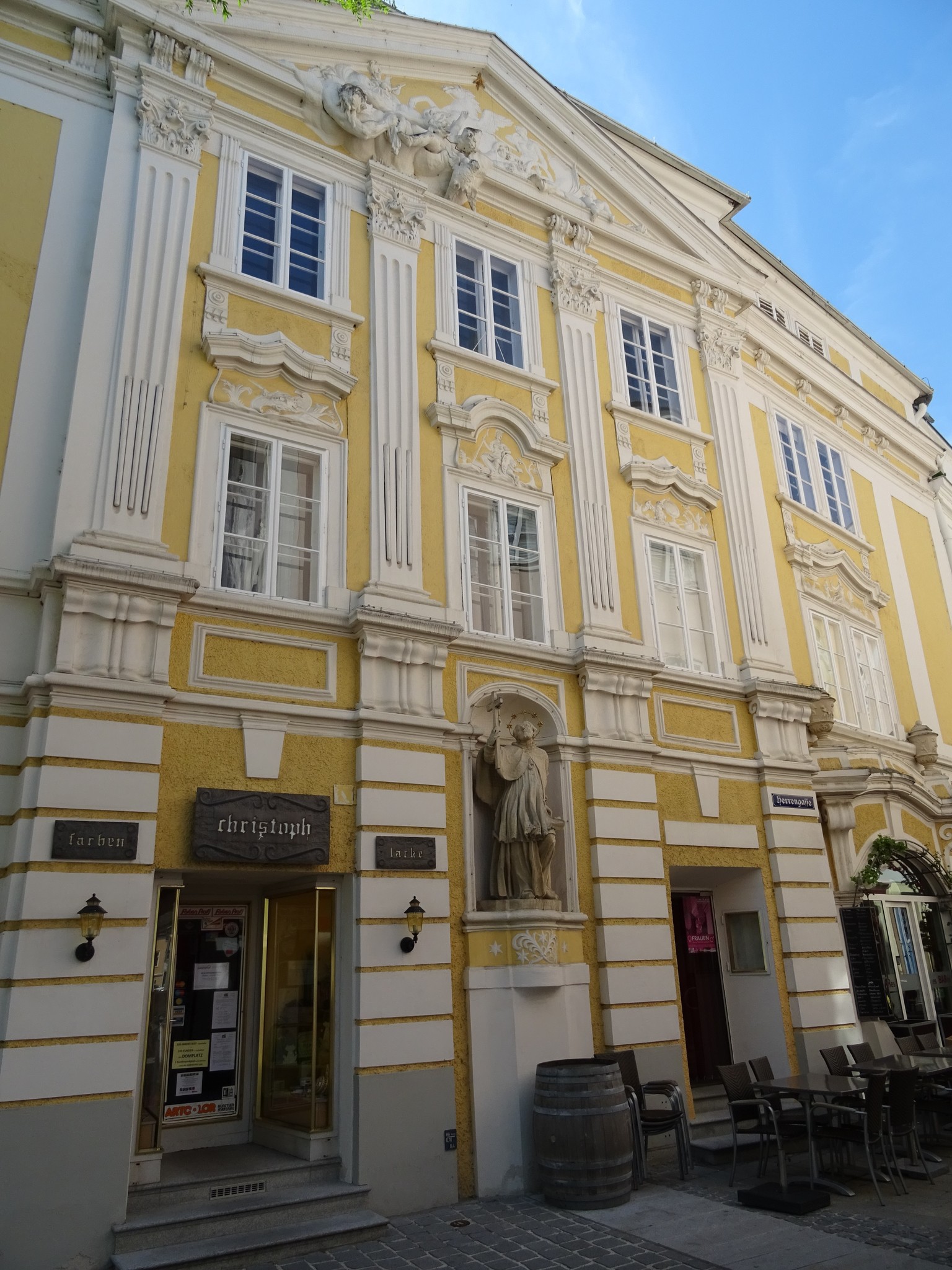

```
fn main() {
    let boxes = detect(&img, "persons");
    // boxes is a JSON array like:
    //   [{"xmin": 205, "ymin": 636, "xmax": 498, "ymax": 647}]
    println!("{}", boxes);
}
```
[{"xmin": 473, "ymin": 720, "xmax": 566, "ymax": 901}]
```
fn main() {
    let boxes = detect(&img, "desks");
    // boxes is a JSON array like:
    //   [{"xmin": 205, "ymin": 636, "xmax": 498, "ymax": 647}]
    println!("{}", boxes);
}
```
[
  {"xmin": 911, "ymin": 1046, "xmax": 952, "ymax": 1057},
  {"xmin": 749, "ymin": 1072, "xmax": 889, "ymax": 1193},
  {"xmin": 841, "ymin": 1054, "xmax": 952, "ymax": 1166}
]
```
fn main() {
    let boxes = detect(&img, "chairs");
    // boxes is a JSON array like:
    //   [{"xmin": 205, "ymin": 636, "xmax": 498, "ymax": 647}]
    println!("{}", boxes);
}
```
[
  {"xmin": 594, "ymin": 1049, "xmax": 694, "ymax": 1189},
  {"xmin": 716, "ymin": 1032, "xmax": 952, "ymax": 1206}
]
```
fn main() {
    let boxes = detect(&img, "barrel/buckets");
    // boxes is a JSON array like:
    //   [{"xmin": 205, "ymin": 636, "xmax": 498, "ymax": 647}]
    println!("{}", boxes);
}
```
[{"xmin": 533, "ymin": 1058, "xmax": 634, "ymax": 1210}]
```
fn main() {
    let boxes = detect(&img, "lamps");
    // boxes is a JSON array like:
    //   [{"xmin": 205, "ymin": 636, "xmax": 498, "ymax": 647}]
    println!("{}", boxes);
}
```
[
  {"xmin": 400, "ymin": 895, "xmax": 426, "ymax": 953},
  {"xmin": 75, "ymin": 893, "xmax": 108, "ymax": 962}
]
